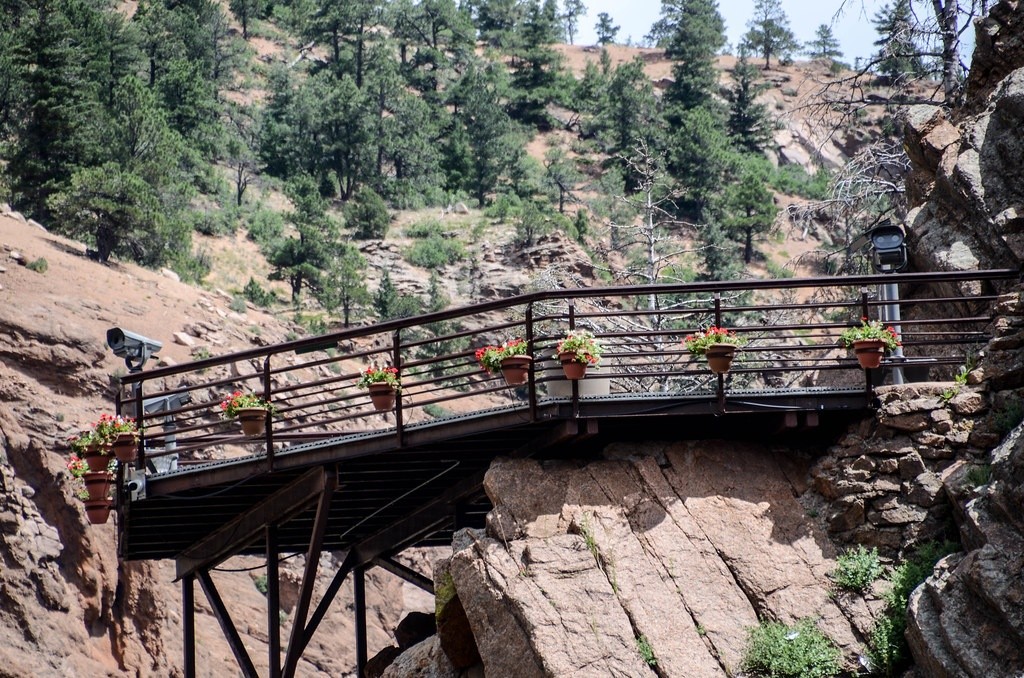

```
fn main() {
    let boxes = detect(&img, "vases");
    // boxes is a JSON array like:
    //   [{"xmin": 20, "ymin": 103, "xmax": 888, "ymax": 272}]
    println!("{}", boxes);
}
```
[
  {"xmin": 109, "ymin": 430, "xmax": 139, "ymax": 462},
  {"xmin": 82, "ymin": 443, "xmax": 112, "ymax": 472},
  {"xmin": 851, "ymin": 338, "xmax": 887, "ymax": 370},
  {"xmin": 704, "ymin": 344, "xmax": 737, "ymax": 374},
  {"xmin": 82, "ymin": 498, "xmax": 112, "ymax": 524},
  {"xmin": 236, "ymin": 408, "xmax": 269, "ymax": 438},
  {"xmin": 557, "ymin": 351, "xmax": 591, "ymax": 381},
  {"xmin": 80, "ymin": 472, "xmax": 113, "ymax": 499},
  {"xmin": 367, "ymin": 381, "xmax": 398, "ymax": 412},
  {"xmin": 500, "ymin": 355, "xmax": 531, "ymax": 386}
]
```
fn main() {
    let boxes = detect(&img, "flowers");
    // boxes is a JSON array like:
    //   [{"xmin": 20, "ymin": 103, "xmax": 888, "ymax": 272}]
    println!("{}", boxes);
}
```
[
  {"xmin": 836, "ymin": 315, "xmax": 904, "ymax": 359},
  {"xmin": 475, "ymin": 335, "xmax": 529, "ymax": 377},
  {"xmin": 355, "ymin": 365, "xmax": 406, "ymax": 396},
  {"xmin": 551, "ymin": 327, "xmax": 604, "ymax": 371},
  {"xmin": 64, "ymin": 428, "xmax": 111, "ymax": 479},
  {"xmin": 680, "ymin": 324, "xmax": 748, "ymax": 363},
  {"xmin": 220, "ymin": 388, "xmax": 280, "ymax": 422},
  {"xmin": 92, "ymin": 413, "xmax": 146, "ymax": 447}
]
